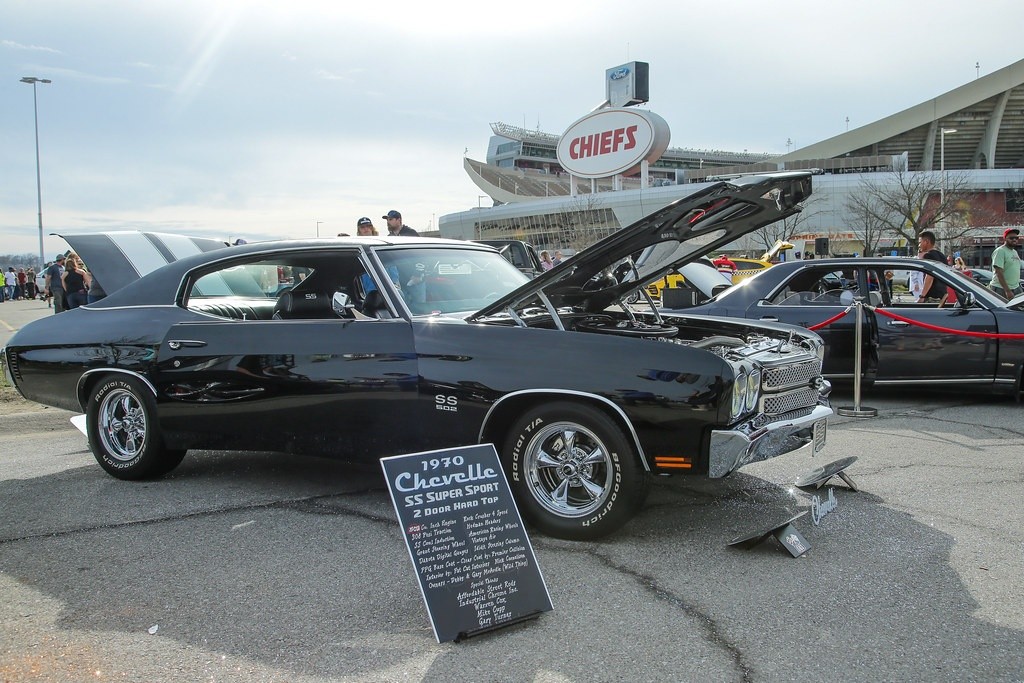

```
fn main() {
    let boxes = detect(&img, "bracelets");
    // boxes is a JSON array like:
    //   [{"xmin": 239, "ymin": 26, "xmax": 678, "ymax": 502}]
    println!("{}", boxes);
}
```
[
  {"xmin": 919, "ymin": 295, "xmax": 925, "ymax": 298},
  {"xmin": 45, "ymin": 286, "xmax": 49, "ymax": 290}
]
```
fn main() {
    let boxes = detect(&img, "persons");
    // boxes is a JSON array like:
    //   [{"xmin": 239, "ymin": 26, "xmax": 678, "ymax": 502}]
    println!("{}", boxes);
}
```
[
  {"xmin": 909, "ymin": 249, "xmax": 926, "ymax": 303},
  {"xmin": 947, "ymin": 255, "xmax": 966, "ymax": 271},
  {"xmin": 0, "ymin": 266, "xmax": 36, "ymax": 302},
  {"xmin": 713, "ymin": 254, "xmax": 736, "ymax": 271},
  {"xmin": 44, "ymin": 251, "xmax": 107, "ymax": 315},
  {"xmin": 382, "ymin": 209, "xmax": 426, "ymax": 306},
  {"xmin": 989, "ymin": 228, "xmax": 1023, "ymax": 302},
  {"xmin": 917, "ymin": 231, "xmax": 948, "ymax": 303},
  {"xmin": 795, "ymin": 251, "xmax": 814, "ymax": 259},
  {"xmin": 224, "ymin": 239, "xmax": 314, "ymax": 295},
  {"xmin": 842, "ymin": 252, "xmax": 894, "ymax": 303},
  {"xmin": 355, "ymin": 215, "xmax": 402, "ymax": 297},
  {"xmin": 539, "ymin": 249, "xmax": 562, "ymax": 271}
]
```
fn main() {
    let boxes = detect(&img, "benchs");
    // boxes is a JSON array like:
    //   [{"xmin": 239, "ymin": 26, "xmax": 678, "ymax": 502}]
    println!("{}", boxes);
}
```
[{"xmin": 189, "ymin": 301, "xmax": 278, "ymax": 320}]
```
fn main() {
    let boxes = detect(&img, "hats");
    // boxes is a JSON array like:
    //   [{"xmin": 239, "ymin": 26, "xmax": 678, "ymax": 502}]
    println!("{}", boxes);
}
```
[
  {"xmin": 1003, "ymin": 228, "xmax": 1020, "ymax": 240},
  {"xmin": 805, "ymin": 251, "xmax": 811, "ymax": 255},
  {"xmin": 358, "ymin": 217, "xmax": 371, "ymax": 227},
  {"xmin": 382, "ymin": 210, "xmax": 401, "ymax": 220},
  {"xmin": 56, "ymin": 254, "xmax": 66, "ymax": 261}
]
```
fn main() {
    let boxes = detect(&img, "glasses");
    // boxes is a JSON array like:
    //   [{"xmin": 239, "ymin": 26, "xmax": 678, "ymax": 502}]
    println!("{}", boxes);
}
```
[
  {"xmin": 78, "ymin": 263, "xmax": 84, "ymax": 265},
  {"xmin": 1006, "ymin": 236, "xmax": 1019, "ymax": 240}
]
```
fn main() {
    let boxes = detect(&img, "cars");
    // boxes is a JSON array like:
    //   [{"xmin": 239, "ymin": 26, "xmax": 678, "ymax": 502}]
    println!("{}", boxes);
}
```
[
  {"xmin": 24, "ymin": 265, "xmax": 65, "ymax": 299},
  {"xmin": 960, "ymin": 268, "xmax": 1024, "ymax": 300},
  {"xmin": 464, "ymin": 236, "xmax": 775, "ymax": 304},
  {"xmin": 873, "ymin": 270, "xmax": 910, "ymax": 289},
  {"xmin": 3, "ymin": 166, "xmax": 833, "ymax": 543},
  {"xmin": 817, "ymin": 270, "xmax": 879, "ymax": 294},
  {"xmin": 643, "ymin": 256, "xmax": 1024, "ymax": 403}
]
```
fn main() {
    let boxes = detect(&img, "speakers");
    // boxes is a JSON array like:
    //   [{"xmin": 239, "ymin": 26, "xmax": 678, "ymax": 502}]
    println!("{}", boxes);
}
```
[{"xmin": 815, "ymin": 238, "xmax": 829, "ymax": 255}]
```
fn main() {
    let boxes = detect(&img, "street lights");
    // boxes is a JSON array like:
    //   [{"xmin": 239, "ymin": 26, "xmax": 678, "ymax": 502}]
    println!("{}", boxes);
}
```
[
  {"xmin": 940, "ymin": 127, "xmax": 957, "ymax": 253},
  {"xmin": 317, "ymin": 221, "xmax": 323, "ymax": 238},
  {"xmin": 19, "ymin": 75, "xmax": 52, "ymax": 271},
  {"xmin": 478, "ymin": 195, "xmax": 489, "ymax": 240}
]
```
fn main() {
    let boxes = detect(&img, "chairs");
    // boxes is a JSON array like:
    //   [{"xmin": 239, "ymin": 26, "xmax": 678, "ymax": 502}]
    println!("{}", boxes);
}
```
[
  {"xmin": 361, "ymin": 290, "xmax": 393, "ymax": 319},
  {"xmin": 272, "ymin": 289, "xmax": 344, "ymax": 319},
  {"xmin": 868, "ymin": 290, "xmax": 885, "ymax": 308}
]
